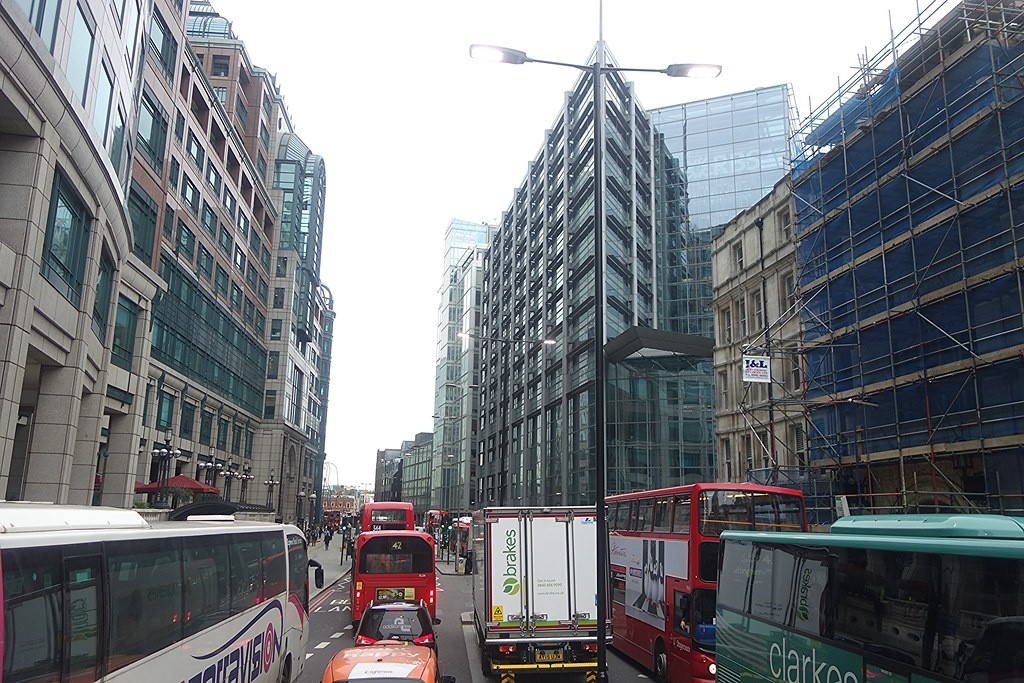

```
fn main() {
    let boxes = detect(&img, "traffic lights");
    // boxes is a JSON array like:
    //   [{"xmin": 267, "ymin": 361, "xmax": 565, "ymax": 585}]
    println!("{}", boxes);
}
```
[{"xmin": 342, "ymin": 517, "xmax": 347, "ymax": 531}]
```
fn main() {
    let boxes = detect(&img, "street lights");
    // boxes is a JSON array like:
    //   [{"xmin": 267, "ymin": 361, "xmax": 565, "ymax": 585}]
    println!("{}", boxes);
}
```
[{"xmin": 467, "ymin": 39, "xmax": 723, "ymax": 683}]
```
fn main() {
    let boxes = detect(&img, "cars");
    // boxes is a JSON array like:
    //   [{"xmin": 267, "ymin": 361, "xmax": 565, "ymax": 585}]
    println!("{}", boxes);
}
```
[
  {"xmin": 352, "ymin": 599, "xmax": 441, "ymax": 663},
  {"xmin": 320, "ymin": 640, "xmax": 457, "ymax": 683}
]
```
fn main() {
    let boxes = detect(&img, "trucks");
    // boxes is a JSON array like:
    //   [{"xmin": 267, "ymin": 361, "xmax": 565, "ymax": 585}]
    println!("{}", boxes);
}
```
[{"xmin": 471, "ymin": 506, "xmax": 609, "ymax": 682}]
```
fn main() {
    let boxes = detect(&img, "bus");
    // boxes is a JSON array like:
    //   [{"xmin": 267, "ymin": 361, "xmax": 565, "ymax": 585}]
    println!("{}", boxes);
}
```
[
  {"xmin": 423, "ymin": 510, "xmax": 452, "ymax": 549},
  {"xmin": 350, "ymin": 531, "xmax": 436, "ymax": 633},
  {"xmin": 0, "ymin": 502, "xmax": 325, "ymax": 683},
  {"xmin": 715, "ymin": 514, "xmax": 1024, "ymax": 683},
  {"xmin": 602, "ymin": 484, "xmax": 811, "ymax": 683},
  {"xmin": 450, "ymin": 517, "xmax": 484, "ymax": 557},
  {"xmin": 322, "ymin": 510, "xmax": 340, "ymax": 531},
  {"xmin": 354, "ymin": 501, "xmax": 415, "ymax": 536}
]
